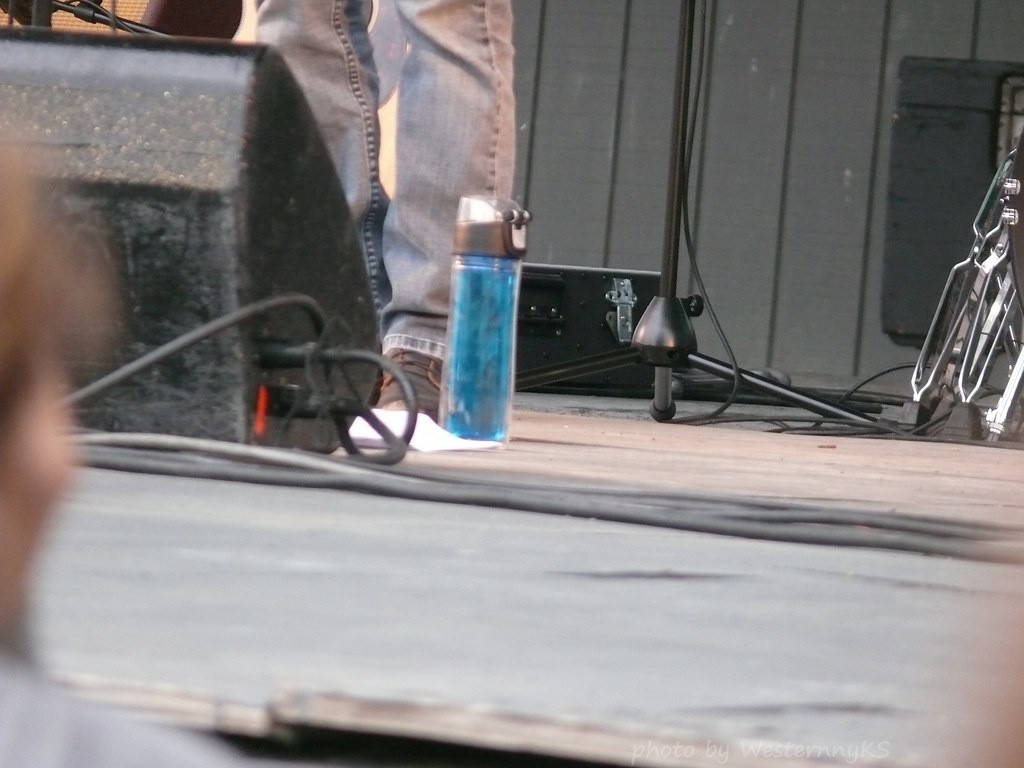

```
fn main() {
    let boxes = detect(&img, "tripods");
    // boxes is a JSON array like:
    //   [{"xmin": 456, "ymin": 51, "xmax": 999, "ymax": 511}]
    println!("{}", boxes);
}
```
[{"xmin": 511, "ymin": 0, "xmax": 895, "ymax": 431}]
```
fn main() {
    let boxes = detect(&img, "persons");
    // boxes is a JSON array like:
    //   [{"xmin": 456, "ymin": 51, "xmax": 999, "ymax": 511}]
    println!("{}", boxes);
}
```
[
  {"xmin": 231, "ymin": 0, "xmax": 516, "ymax": 426},
  {"xmin": 0, "ymin": 109, "xmax": 257, "ymax": 768}
]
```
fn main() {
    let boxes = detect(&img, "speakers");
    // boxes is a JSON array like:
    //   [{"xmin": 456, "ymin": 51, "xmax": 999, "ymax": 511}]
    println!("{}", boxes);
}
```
[
  {"xmin": 881, "ymin": 54, "xmax": 1024, "ymax": 347},
  {"xmin": 0, "ymin": 25, "xmax": 383, "ymax": 455},
  {"xmin": 511, "ymin": 262, "xmax": 663, "ymax": 399}
]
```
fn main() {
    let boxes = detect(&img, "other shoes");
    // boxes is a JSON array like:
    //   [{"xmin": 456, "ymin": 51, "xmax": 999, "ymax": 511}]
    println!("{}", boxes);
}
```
[{"xmin": 376, "ymin": 348, "xmax": 443, "ymax": 424}]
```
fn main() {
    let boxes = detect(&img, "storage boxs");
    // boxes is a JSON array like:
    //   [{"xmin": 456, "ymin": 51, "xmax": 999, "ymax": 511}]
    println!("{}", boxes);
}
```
[{"xmin": 514, "ymin": 263, "xmax": 659, "ymax": 399}]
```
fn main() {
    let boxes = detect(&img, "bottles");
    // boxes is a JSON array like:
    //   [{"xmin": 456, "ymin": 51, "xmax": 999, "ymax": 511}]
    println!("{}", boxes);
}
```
[{"xmin": 442, "ymin": 194, "xmax": 533, "ymax": 442}]
